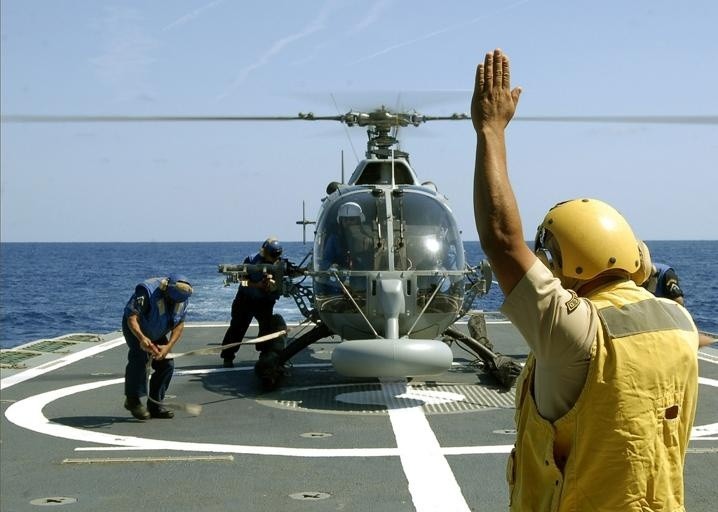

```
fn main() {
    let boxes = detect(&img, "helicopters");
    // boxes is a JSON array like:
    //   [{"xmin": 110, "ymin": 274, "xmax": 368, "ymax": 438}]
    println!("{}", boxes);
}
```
[{"xmin": 0, "ymin": 88, "xmax": 717, "ymax": 391}]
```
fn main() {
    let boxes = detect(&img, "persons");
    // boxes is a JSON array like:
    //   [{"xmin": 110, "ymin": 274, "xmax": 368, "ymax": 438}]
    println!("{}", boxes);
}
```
[
  {"xmin": 219, "ymin": 236, "xmax": 295, "ymax": 366},
  {"xmin": 468, "ymin": 47, "xmax": 701, "ymax": 511},
  {"xmin": 120, "ymin": 275, "xmax": 194, "ymax": 419},
  {"xmin": 418, "ymin": 222, "xmax": 455, "ymax": 295},
  {"xmin": 322, "ymin": 202, "xmax": 378, "ymax": 283},
  {"xmin": 643, "ymin": 257, "xmax": 684, "ymax": 306}
]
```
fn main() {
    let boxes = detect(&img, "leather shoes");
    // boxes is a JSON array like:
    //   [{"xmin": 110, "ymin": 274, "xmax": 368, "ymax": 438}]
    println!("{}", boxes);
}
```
[
  {"xmin": 147, "ymin": 398, "xmax": 174, "ymax": 418},
  {"xmin": 124, "ymin": 397, "xmax": 150, "ymax": 420}
]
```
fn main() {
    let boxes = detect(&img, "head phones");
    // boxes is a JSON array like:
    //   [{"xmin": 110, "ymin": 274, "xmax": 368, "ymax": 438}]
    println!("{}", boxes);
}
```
[
  {"xmin": 336, "ymin": 201, "xmax": 366, "ymax": 224},
  {"xmin": 260, "ymin": 239, "xmax": 272, "ymax": 257},
  {"xmin": 159, "ymin": 278, "xmax": 193, "ymax": 293},
  {"xmin": 535, "ymin": 199, "xmax": 651, "ymax": 285}
]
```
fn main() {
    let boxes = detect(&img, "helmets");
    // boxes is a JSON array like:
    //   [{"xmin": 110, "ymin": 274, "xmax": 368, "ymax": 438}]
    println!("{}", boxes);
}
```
[
  {"xmin": 533, "ymin": 196, "xmax": 639, "ymax": 284},
  {"xmin": 261, "ymin": 238, "xmax": 282, "ymax": 261},
  {"xmin": 160, "ymin": 274, "xmax": 191, "ymax": 302}
]
